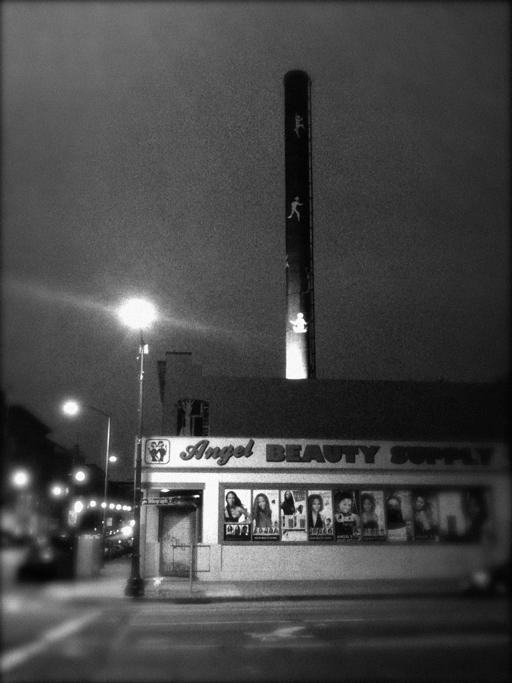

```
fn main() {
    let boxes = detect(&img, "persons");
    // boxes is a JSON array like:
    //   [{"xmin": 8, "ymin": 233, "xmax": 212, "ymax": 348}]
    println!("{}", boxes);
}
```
[{"xmin": 224, "ymin": 490, "xmax": 434, "ymax": 542}]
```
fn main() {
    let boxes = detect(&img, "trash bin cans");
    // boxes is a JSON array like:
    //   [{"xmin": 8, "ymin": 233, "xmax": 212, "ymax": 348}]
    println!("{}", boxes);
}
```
[{"xmin": 75, "ymin": 533, "xmax": 104, "ymax": 580}]
